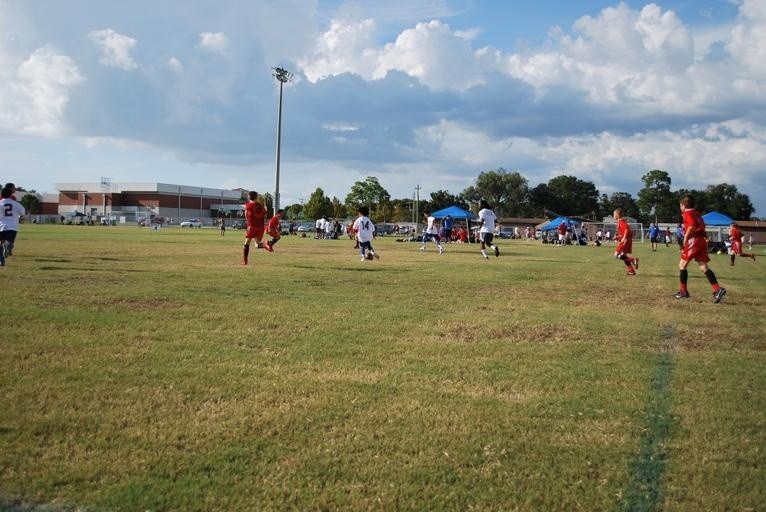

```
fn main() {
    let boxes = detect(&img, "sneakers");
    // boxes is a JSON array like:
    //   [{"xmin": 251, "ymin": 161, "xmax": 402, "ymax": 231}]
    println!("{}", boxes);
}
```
[
  {"xmin": 627, "ymin": 271, "xmax": 635, "ymax": 275},
  {"xmin": 673, "ymin": 291, "xmax": 689, "ymax": 299},
  {"xmin": 634, "ymin": 257, "xmax": 638, "ymax": 270},
  {"xmin": 713, "ymin": 288, "xmax": 726, "ymax": 303},
  {"xmin": 495, "ymin": 246, "xmax": 499, "ymax": 257}
]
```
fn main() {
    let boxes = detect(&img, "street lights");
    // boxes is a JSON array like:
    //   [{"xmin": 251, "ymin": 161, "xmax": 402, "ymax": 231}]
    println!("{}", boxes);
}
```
[{"xmin": 268, "ymin": 65, "xmax": 294, "ymax": 217}]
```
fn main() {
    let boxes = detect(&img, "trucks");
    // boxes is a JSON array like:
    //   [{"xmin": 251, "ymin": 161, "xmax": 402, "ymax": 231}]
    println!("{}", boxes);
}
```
[{"xmin": 598, "ymin": 217, "xmax": 638, "ymax": 241}]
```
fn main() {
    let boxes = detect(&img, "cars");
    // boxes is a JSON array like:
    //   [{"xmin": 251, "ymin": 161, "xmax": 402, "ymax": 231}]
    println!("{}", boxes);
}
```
[
  {"xmin": 653, "ymin": 229, "xmax": 673, "ymax": 245},
  {"xmin": 470, "ymin": 224, "xmax": 480, "ymax": 232},
  {"xmin": 180, "ymin": 218, "xmax": 201, "ymax": 228},
  {"xmin": 139, "ymin": 214, "xmax": 164, "ymax": 227},
  {"xmin": 535, "ymin": 227, "xmax": 546, "ymax": 240},
  {"xmin": 230, "ymin": 218, "xmax": 414, "ymax": 235},
  {"xmin": 500, "ymin": 227, "xmax": 515, "ymax": 239}
]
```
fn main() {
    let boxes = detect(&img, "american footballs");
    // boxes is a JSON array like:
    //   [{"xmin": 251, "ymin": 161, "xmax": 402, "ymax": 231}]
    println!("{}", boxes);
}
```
[{"xmin": 367, "ymin": 253, "xmax": 373, "ymax": 260}]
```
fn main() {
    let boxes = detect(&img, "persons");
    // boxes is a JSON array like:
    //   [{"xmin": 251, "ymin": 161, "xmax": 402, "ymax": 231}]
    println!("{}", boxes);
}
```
[
  {"xmin": 0, "ymin": 188, "xmax": 26, "ymax": 266},
  {"xmin": 263, "ymin": 209, "xmax": 286, "ymax": 252},
  {"xmin": 673, "ymin": 197, "xmax": 727, "ymax": 304},
  {"xmin": 279, "ymin": 220, "xmax": 299, "ymax": 236},
  {"xmin": 219, "ymin": 216, "xmax": 239, "ymax": 236},
  {"xmin": 166, "ymin": 215, "xmax": 173, "ymax": 226},
  {"xmin": 314, "ymin": 207, "xmax": 380, "ymax": 261},
  {"xmin": 645, "ymin": 222, "xmax": 755, "ymax": 267},
  {"xmin": 492, "ymin": 219, "xmax": 611, "ymax": 246},
  {"xmin": 0, "ymin": 183, "xmax": 16, "ymax": 258},
  {"xmin": 240, "ymin": 191, "xmax": 273, "ymax": 266},
  {"xmin": 392, "ymin": 209, "xmax": 478, "ymax": 254},
  {"xmin": 476, "ymin": 200, "xmax": 500, "ymax": 260},
  {"xmin": 613, "ymin": 208, "xmax": 639, "ymax": 275}
]
